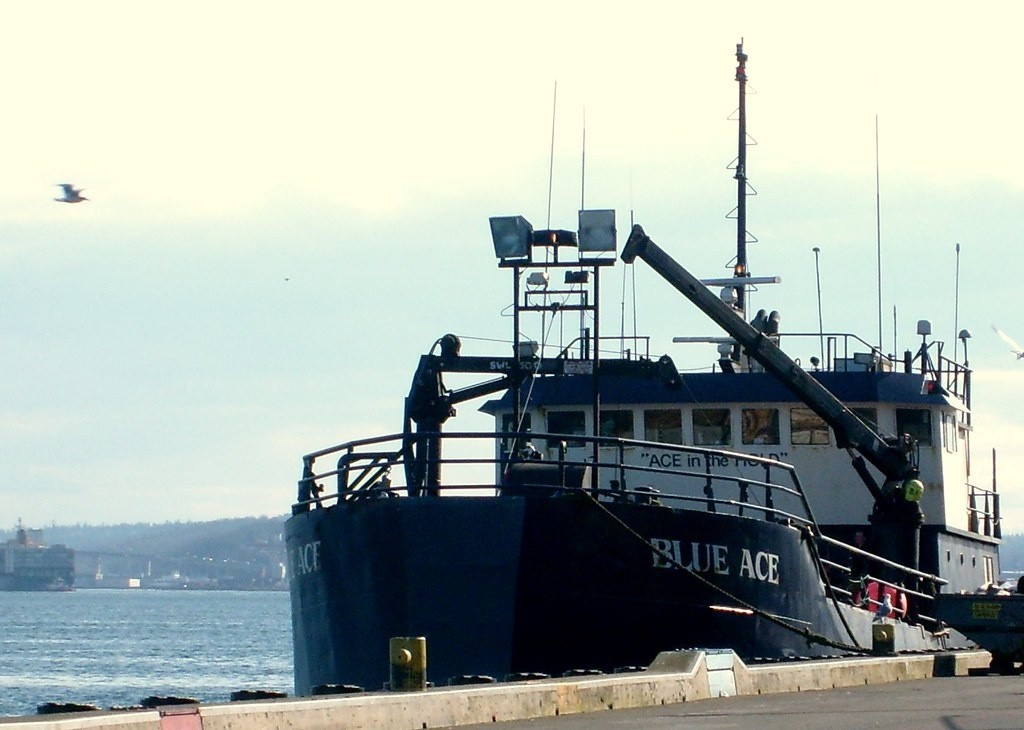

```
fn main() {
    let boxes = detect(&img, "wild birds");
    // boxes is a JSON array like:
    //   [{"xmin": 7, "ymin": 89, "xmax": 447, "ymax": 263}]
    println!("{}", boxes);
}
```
[{"xmin": 53, "ymin": 183, "xmax": 88, "ymax": 203}]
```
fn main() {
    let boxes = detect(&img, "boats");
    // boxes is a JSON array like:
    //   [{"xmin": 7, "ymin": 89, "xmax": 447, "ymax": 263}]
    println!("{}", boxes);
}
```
[{"xmin": 285, "ymin": 34, "xmax": 1024, "ymax": 695}]
